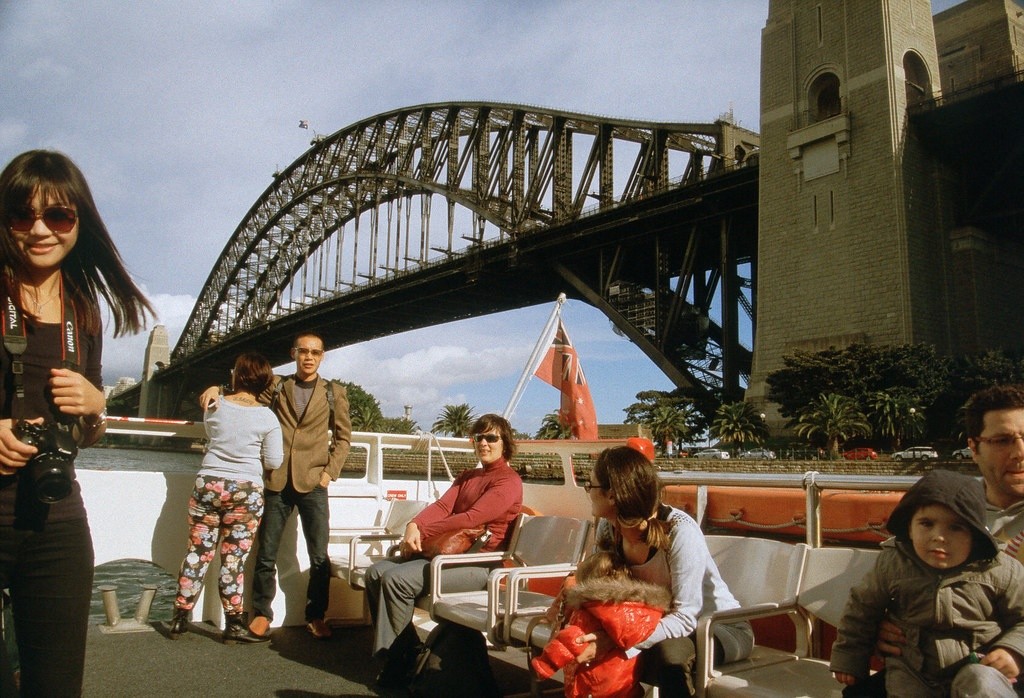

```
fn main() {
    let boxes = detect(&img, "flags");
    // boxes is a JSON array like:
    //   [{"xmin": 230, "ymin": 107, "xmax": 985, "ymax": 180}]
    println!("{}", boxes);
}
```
[{"xmin": 531, "ymin": 314, "xmax": 599, "ymax": 441}]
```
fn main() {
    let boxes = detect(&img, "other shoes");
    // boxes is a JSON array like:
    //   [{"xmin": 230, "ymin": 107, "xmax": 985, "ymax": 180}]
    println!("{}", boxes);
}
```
[
  {"xmin": 376, "ymin": 645, "xmax": 421, "ymax": 687},
  {"xmin": 250, "ymin": 615, "xmax": 272, "ymax": 637},
  {"xmin": 306, "ymin": 620, "xmax": 330, "ymax": 639}
]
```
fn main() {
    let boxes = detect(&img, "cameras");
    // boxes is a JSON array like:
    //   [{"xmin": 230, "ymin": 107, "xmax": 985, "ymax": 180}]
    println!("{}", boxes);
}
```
[{"xmin": 10, "ymin": 420, "xmax": 78, "ymax": 504}]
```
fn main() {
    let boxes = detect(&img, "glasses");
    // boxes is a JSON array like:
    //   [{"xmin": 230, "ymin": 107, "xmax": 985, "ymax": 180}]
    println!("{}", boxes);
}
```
[
  {"xmin": 473, "ymin": 432, "xmax": 503, "ymax": 444},
  {"xmin": 294, "ymin": 348, "xmax": 324, "ymax": 357},
  {"xmin": 972, "ymin": 435, "xmax": 1024, "ymax": 452},
  {"xmin": 230, "ymin": 368, "xmax": 237, "ymax": 375},
  {"xmin": 6, "ymin": 206, "xmax": 79, "ymax": 233},
  {"xmin": 584, "ymin": 480, "xmax": 609, "ymax": 494}
]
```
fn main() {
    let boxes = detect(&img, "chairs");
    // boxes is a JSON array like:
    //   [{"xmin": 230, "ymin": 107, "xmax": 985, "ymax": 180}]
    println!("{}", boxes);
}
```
[{"xmin": 329, "ymin": 498, "xmax": 887, "ymax": 698}]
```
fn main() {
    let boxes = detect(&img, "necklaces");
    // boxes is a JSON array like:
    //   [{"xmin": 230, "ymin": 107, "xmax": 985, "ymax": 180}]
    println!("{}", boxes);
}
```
[{"xmin": 24, "ymin": 289, "xmax": 60, "ymax": 314}]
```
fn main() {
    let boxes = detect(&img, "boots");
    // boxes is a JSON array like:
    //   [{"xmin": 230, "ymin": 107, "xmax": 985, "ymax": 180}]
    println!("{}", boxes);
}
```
[
  {"xmin": 223, "ymin": 613, "xmax": 271, "ymax": 645},
  {"xmin": 170, "ymin": 607, "xmax": 189, "ymax": 641}
]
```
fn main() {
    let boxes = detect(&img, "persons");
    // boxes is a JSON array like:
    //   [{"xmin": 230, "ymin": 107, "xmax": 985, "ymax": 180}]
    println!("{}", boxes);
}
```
[
  {"xmin": 168, "ymin": 350, "xmax": 285, "ymax": 644},
  {"xmin": 199, "ymin": 333, "xmax": 353, "ymax": 638},
  {"xmin": 528, "ymin": 445, "xmax": 755, "ymax": 698},
  {"xmin": 828, "ymin": 385, "xmax": 1024, "ymax": 698},
  {"xmin": 365, "ymin": 414, "xmax": 523, "ymax": 686},
  {"xmin": 1, "ymin": 149, "xmax": 162, "ymax": 698}
]
